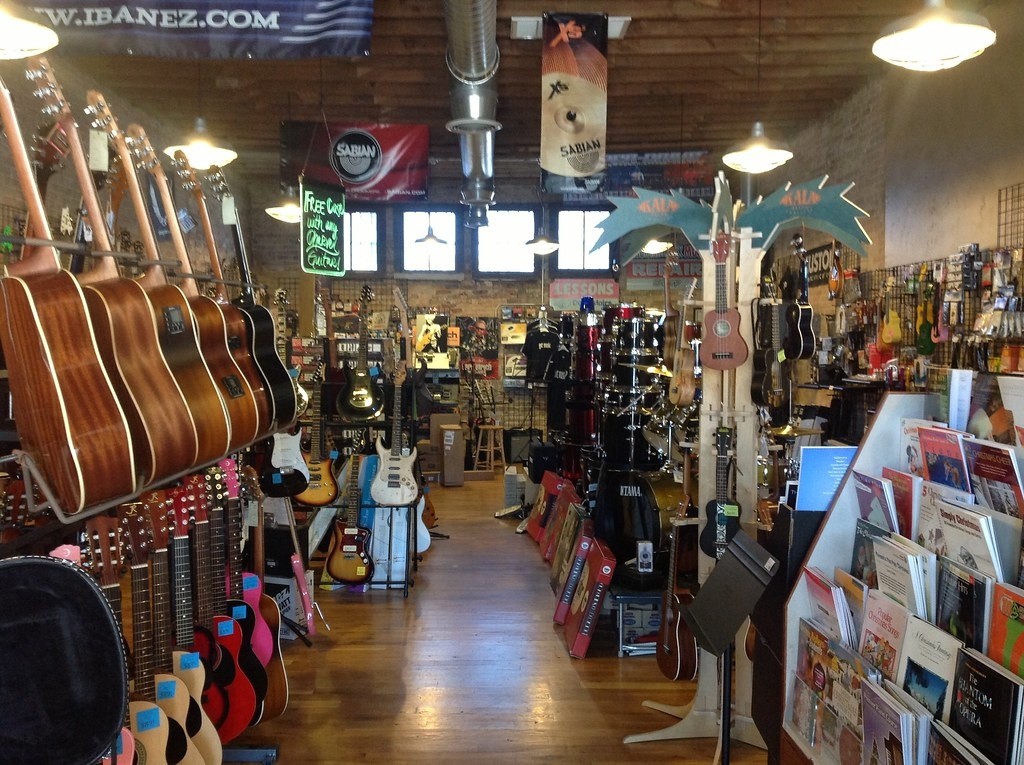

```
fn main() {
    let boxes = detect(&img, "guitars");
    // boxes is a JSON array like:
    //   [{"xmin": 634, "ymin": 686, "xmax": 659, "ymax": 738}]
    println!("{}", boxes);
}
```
[{"xmin": 0, "ymin": 0, "xmax": 1024, "ymax": 765}]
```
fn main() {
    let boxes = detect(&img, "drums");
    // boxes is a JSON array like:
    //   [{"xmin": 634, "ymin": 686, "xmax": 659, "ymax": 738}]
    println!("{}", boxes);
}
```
[
  {"xmin": 573, "ymin": 302, "xmax": 675, "ymax": 471},
  {"xmin": 594, "ymin": 463, "xmax": 687, "ymax": 551}
]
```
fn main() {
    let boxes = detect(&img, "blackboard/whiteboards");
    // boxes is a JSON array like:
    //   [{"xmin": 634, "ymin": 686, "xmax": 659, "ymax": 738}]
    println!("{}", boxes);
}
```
[{"xmin": 298, "ymin": 175, "xmax": 347, "ymax": 278}]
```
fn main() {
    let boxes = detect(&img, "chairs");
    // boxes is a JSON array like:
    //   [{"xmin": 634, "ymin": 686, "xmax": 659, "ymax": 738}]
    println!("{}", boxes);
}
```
[{"xmin": 0, "ymin": 556, "xmax": 130, "ymax": 765}]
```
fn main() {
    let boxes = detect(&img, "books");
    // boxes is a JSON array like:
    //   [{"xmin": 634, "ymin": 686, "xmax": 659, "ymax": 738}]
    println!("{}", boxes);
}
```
[
  {"xmin": 792, "ymin": 616, "xmax": 994, "ymax": 765},
  {"xmin": 850, "ymin": 466, "xmax": 1024, "ymax": 583},
  {"xmin": 851, "ymin": 516, "xmax": 1024, "ymax": 678},
  {"xmin": 802, "ymin": 564, "xmax": 1024, "ymax": 765},
  {"xmin": 899, "ymin": 417, "xmax": 1024, "ymax": 519},
  {"xmin": 915, "ymin": 366, "xmax": 1024, "ymax": 448}
]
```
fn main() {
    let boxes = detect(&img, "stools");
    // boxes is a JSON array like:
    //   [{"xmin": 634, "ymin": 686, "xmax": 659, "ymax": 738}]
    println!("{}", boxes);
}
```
[{"xmin": 473, "ymin": 425, "xmax": 507, "ymax": 472}]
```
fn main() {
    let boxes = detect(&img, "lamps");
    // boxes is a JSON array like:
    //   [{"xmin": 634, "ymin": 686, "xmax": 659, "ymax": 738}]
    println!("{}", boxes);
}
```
[
  {"xmin": 415, "ymin": 212, "xmax": 448, "ymax": 247},
  {"xmin": 264, "ymin": 93, "xmax": 302, "ymax": 224},
  {"xmin": 525, "ymin": 169, "xmax": 561, "ymax": 255},
  {"xmin": 872, "ymin": 0, "xmax": 997, "ymax": 70},
  {"xmin": 162, "ymin": 0, "xmax": 239, "ymax": 170},
  {"xmin": 0, "ymin": 1, "xmax": 62, "ymax": 61},
  {"xmin": 721, "ymin": 1, "xmax": 795, "ymax": 174}
]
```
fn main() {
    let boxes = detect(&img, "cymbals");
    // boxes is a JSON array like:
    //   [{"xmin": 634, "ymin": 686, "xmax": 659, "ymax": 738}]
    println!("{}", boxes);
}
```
[
  {"xmin": 769, "ymin": 425, "xmax": 826, "ymax": 440},
  {"xmin": 618, "ymin": 363, "xmax": 674, "ymax": 377}
]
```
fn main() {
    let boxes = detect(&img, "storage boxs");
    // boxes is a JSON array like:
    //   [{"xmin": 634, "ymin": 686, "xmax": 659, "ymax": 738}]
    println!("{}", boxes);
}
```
[
  {"xmin": 503, "ymin": 464, "xmax": 660, "ymax": 660},
  {"xmin": 263, "ymin": 571, "xmax": 315, "ymax": 640},
  {"xmin": 307, "ymin": 453, "xmax": 408, "ymax": 592},
  {"xmin": 499, "ymin": 322, "xmax": 527, "ymax": 387},
  {"xmin": 246, "ymin": 522, "xmax": 309, "ymax": 578},
  {"xmin": 416, "ymin": 425, "xmax": 464, "ymax": 487}
]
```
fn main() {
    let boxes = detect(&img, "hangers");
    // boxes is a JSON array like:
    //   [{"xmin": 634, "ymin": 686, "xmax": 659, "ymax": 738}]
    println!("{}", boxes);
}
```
[{"xmin": 537, "ymin": 307, "xmax": 549, "ymax": 332}]
